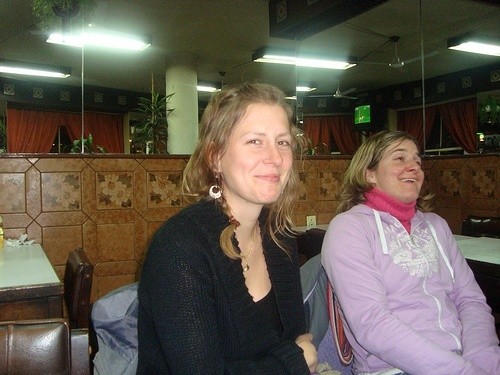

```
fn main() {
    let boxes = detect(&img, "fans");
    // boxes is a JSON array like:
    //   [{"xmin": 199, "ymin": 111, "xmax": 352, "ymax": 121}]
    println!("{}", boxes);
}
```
[
  {"xmin": 305, "ymin": 74, "xmax": 359, "ymax": 100},
  {"xmin": 344, "ymin": 36, "xmax": 441, "ymax": 78}
]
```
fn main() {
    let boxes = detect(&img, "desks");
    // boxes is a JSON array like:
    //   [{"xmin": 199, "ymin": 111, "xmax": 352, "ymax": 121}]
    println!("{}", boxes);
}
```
[
  {"xmin": 0, "ymin": 243, "xmax": 64, "ymax": 322},
  {"xmin": 452, "ymin": 234, "xmax": 500, "ymax": 345},
  {"xmin": 289, "ymin": 224, "xmax": 330, "ymax": 255}
]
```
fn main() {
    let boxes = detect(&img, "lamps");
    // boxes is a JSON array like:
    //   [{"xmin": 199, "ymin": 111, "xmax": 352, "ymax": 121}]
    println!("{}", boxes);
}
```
[
  {"xmin": 252, "ymin": 46, "xmax": 358, "ymax": 70},
  {"xmin": 0, "ymin": 59, "xmax": 73, "ymax": 81}
]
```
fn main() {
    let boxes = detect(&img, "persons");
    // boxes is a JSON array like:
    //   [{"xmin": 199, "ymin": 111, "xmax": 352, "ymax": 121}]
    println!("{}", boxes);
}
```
[
  {"xmin": 136, "ymin": 81, "xmax": 317, "ymax": 375},
  {"xmin": 320, "ymin": 131, "xmax": 500, "ymax": 375}
]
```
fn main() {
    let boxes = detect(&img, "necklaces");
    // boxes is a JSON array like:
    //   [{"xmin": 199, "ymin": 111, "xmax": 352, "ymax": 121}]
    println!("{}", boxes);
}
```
[{"xmin": 238, "ymin": 226, "xmax": 259, "ymax": 271}]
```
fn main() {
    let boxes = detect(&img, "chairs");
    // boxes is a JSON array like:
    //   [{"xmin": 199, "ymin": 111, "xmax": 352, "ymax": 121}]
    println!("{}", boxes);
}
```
[{"xmin": 0, "ymin": 217, "xmax": 500, "ymax": 375}]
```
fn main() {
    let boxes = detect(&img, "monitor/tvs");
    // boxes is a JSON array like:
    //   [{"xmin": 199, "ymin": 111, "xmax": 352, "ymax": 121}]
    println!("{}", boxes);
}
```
[{"xmin": 354, "ymin": 104, "xmax": 371, "ymax": 125}]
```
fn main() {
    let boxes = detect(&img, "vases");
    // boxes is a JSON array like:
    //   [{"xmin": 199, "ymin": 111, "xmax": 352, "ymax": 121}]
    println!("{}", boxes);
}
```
[{"xmin": 146, "ymin": 143, "xmax": 154, "ymax": 154}]
